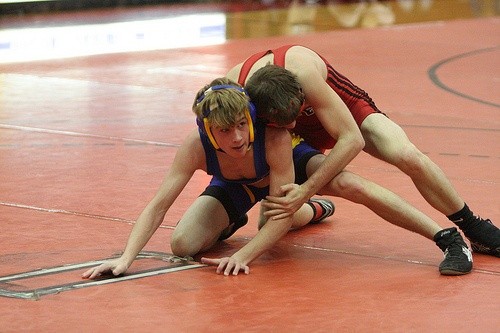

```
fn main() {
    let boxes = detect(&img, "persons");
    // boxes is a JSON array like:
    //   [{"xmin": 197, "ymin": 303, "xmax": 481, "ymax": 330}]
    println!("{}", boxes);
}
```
[
  {"xmin": 81, "ymin": 77, "xmax": 474, "ymax": 281},
  {"xmin": 225, "ymin": 42, "xmax": 500, "ymax": 256}
]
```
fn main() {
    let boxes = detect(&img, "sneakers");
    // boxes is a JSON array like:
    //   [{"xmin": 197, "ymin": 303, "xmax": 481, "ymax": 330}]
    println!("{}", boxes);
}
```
[
  {"xmin": 457, "ymin": 214, "xmax": 500, "ymax": 257},
  {"xmin": 308, "ymin": 197, "xmax": 335, "ymax": 225},
  {"xmin": 438, "ymin": 231, "xmax": 474, "ymax": 276}
]
